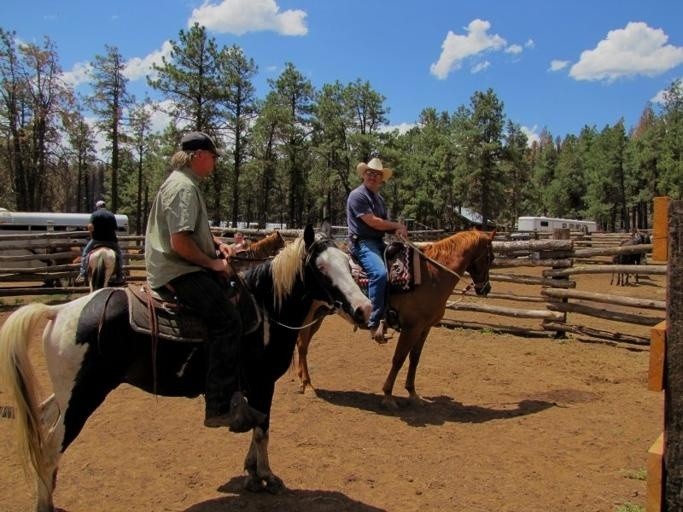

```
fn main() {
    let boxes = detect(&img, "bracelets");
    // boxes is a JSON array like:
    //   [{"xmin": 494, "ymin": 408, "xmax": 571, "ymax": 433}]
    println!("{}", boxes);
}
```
[{"xmin": 215, "ymin": 241, "xmax": 225, "ymax": 250}]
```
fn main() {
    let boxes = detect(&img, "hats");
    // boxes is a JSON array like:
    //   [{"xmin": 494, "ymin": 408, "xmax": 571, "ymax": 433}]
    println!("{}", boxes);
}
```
[
  {"xmin": 96, "ymin": 200, "xmax": 106, "ymax": 208},
  {"xmin": 182, "ymin": 131, "xmax": 221, "ymax": 157},
  {"xmin": 357, "ymin": 157, "xmax": 393, "ymax": 182}
]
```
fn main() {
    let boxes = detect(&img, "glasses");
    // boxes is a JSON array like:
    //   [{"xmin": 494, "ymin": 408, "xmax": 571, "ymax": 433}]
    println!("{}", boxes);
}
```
[{"xmin": 365, "ymin": 169, "xmax": 382, "ymax": 178}]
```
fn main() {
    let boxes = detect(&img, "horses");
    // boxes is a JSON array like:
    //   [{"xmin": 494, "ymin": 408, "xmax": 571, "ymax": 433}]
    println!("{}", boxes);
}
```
[
  {"xmin": 230, "ymin": 231, "xmax": 287, "ymax": 272},
  {"xmin": 610, "ymin": 231, "xmax": 652, "ymax": 286},
  {"xmin": 0, "ymin": 216, "xmax": 373, "ymax": 512},
  {"xmin": 85, "ymin": 246, "xmax": 116, "ymax": 293},
  {"xmin": 288, "ymin": 226, "xmax": 496, "ymax": 412}
]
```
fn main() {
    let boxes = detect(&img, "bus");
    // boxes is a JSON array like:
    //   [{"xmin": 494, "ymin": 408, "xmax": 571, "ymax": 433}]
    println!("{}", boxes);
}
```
[
  {"xmin": 0, "ymin": 211, "xmax": 130, "ymax": 277},
  {"xmin": 519, "ymin": 217, "xmax": 597, "ymax": 232}
]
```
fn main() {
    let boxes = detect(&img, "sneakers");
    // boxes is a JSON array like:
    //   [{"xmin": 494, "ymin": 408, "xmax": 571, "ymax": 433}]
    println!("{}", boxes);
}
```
[
  {"xmin": 371, "ymin": 326, "xmax": 393, "ymax": 340},
  {"xmin": 204, "ymin": 403, "xmax": 259, "ymax": 433},
  {"xmin": 74, "ymin": 276, "xmax": 84, "ymax": 282}
]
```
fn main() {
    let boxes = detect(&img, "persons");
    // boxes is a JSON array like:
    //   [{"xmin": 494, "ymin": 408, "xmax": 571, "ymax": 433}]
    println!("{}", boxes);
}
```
[
  {"xmin": 230, "ymin": 231, "xmax": 247, "ymax": 252},
  {"xmin": 344, "ymin": 155, "xmax": 407, "ymax": 344},
  {"xmin": 141, "ymin": 130, "xmax": 270, "ymax": 432},
  {"xmin": 72, "ymin": 200, "xmax": 126, "ymax": 286}
]
